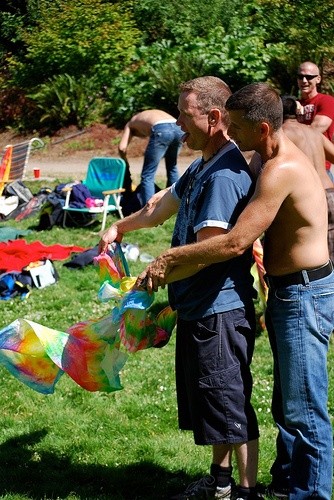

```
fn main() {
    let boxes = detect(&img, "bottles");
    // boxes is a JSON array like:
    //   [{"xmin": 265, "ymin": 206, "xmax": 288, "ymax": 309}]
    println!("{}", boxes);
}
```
[{"xmin": 123, "ymin": 243, "xmax": 139, "ymax": 259}]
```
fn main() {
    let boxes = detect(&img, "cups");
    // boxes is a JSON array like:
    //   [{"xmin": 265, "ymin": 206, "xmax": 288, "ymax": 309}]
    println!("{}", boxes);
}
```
[{"xmin": 33, "ymin": 169, "xmax": 40, "ymax": 178}]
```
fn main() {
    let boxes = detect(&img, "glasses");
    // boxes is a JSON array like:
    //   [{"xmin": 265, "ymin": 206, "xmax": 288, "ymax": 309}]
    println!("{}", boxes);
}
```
[{"xmin": 297, "ymin": 74, "xmax": 318, "ymax": 80}]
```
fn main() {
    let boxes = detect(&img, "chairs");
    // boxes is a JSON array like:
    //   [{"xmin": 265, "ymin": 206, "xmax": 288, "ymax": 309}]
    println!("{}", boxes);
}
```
[
  {"xmin": 62, "ymin": 157, "xmax": 127, "ymax": 235},
  {"xmin": 0, "ymin": 137, "xmax": 44, "ymax": 196}
]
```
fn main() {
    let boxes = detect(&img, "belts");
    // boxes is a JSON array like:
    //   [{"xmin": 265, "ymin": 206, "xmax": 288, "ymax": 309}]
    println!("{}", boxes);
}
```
[{"xmin": 263, "ymin": 260, "xmax": 333, "ymax": 290}]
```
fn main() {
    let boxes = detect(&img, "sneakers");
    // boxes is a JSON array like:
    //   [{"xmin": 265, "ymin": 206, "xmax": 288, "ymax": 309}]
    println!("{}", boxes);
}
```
[
  {"xmin": 230, "ymin": 487, "xmax": 264, "ymax": 500},
  {"xmin": 256, "ymin": 482, "xmax": 292, "ymax": 500},
  {"xmin": 171, "ymin": 476, "xmax": 236, "ymax": 500}
]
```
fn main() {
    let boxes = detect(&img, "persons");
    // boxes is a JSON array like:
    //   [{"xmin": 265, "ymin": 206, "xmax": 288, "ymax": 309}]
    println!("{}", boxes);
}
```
[
  {"xmin": 95, "ymin": 60, "xmax": 334, "ymax": 500},
  {"xmin": 117, "ymin": 108, "xmax": 187, "ymax": 202}
]
